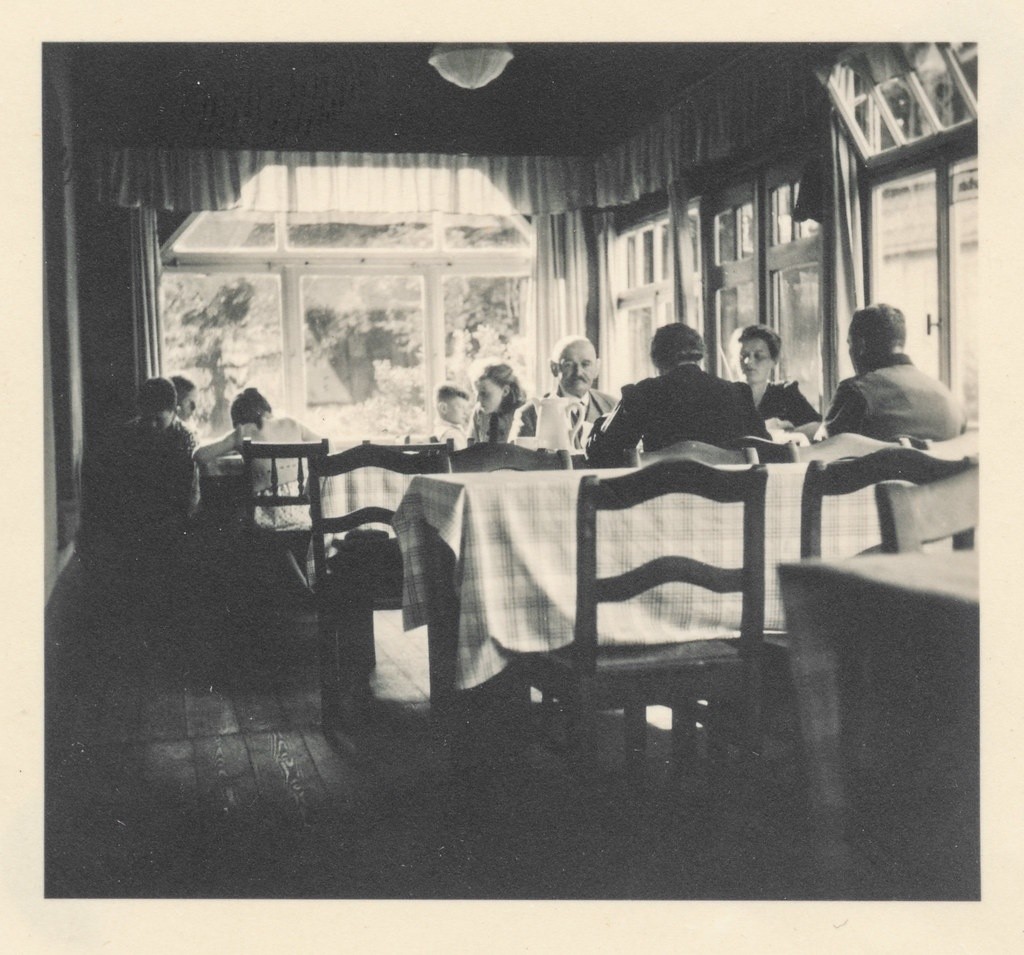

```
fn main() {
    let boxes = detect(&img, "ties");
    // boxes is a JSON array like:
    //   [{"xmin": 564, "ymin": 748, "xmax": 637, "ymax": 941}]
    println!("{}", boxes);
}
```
[{"xmin": 575, "ymin": 401, "xmax": 585, "ymax": 419}]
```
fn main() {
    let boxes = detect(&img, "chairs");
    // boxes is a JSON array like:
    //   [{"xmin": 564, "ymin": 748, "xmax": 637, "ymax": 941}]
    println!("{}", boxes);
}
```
[{"xmin": 238, "ymin": 434, "xmax": 979, "ymax": 900}]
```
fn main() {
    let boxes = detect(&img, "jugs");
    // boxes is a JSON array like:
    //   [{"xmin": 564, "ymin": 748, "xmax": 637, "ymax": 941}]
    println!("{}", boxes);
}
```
[{"xmin": 531, "ymin": 396, "xmax": 585, "ymax": 453}]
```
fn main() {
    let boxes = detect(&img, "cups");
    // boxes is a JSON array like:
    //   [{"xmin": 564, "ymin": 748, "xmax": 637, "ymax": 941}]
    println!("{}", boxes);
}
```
[{"xmin": 514, "ymin": 437, "xmax": 538, "ymax": 451}]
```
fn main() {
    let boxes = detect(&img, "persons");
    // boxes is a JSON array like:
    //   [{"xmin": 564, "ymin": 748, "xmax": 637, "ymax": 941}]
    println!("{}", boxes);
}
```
[
  {"xmin": 118, "ymin": 378, "xmax": 199, "ymax": 515},
  {"xmin": 174, "ymin": 376, "xmax": 198, "ymax": 421},
  {"xmin": 508, "ymin": 335, "xmax": 618, "ymax": 451},
  {"xmin": 470, "ymin": 363, "xmax": 526, "ymax": 444},
  {"xmin": 190, "ymin": 388, "xmax": 324, "ymax": 534},
  {"xmin": 813, "ymin": 303, "xmax": 968, "ymax": 443},
  {"xmin": 732, "ymin": 324, "xmax": 823, "ymax": 432},
  {"xmin": 434, "ymin": 383, "xmax": 471, "ymax": 451},
  {"xmin": 580, "ymin": 323, "xmax": 772, "ymax": 466}
]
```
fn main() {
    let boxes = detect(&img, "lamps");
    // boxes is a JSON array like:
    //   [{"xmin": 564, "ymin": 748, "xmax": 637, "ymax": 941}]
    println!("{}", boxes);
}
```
[{"xmin": 428, "ymin": 43, "xmax": 515, "ymax": 91}]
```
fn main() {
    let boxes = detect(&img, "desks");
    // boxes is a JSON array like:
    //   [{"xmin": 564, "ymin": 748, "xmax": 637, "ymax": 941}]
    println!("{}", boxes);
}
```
[
  {"xmin": 195, "ymin": 442, "xmax": 444, "ymax": 590},
  {"xmin": 775, "ymin": 544, "xmax": 980, "ymax": 901},
  {"xmin": 392, "ymin": 462, "xmax": 956, "ymax": 854}
]
